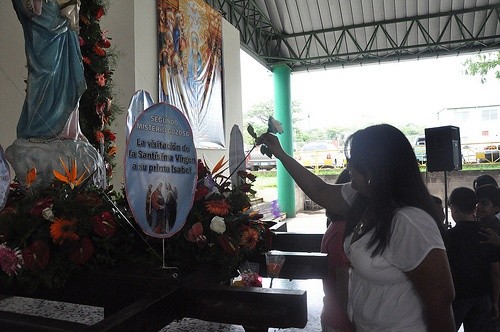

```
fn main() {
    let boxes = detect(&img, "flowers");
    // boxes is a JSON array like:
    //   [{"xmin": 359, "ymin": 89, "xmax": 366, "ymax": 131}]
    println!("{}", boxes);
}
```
[
  {"xmin": 77, "ymin": 0, "xmax": 126, "ymax": 187},
  {"xmin": 0, "ymin": 115, "xmax": 285, "ymax": 288}
]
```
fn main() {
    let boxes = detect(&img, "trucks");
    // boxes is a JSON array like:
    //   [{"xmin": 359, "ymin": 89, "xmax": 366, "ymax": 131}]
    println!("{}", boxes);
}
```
[{"xmin": 244, "ymin": 151, "xmax": 277, "ymax": 171}]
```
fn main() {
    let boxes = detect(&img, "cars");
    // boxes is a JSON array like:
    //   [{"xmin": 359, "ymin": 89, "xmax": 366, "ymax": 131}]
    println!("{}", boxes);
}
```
[
  {"xmin": 297, "ymin": 141, "xmax": 344, "ymax": 168},
  {"xmin": 413, "ymin": 137, "xmax": 500, "ymax": 164}
]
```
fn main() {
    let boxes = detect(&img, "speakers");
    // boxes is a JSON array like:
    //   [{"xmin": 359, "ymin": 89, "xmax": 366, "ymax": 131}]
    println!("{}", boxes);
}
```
[{"xmin": 425, "ymin": 126, "xmax": 462, "ymax": 172}]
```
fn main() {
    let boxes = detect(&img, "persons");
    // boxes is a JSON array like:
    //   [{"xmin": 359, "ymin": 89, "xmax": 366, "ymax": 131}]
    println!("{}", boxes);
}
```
[
  {"xmin": 11, "ymin": 0, "xmax": 87, "ymax": 142},
  {"xmin": 257, "ymin": 123, "xmax": 456, "ymax": 332},
  {"xmin": 321, "ymin": 168, "xmax": 356, "ymax": 332},
  {"xmin": 448, "ymin": 187, "xmax": 500, "ymax": 332},
  {"xmin": 428, "ymin": 194, "xmax": 449, "ymax": 240},
  {"xmin": 474, "ymin": 175, "xmax": 500, "ymax": 247}
]
